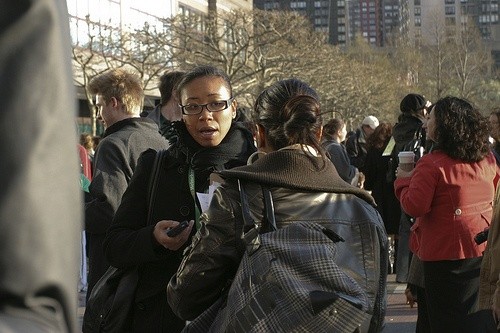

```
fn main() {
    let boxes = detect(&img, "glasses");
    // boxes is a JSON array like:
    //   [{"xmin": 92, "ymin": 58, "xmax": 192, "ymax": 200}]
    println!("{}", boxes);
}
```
[{"xmin": 178, "ymin": 97, "xmax": 234, "ymax": 115}]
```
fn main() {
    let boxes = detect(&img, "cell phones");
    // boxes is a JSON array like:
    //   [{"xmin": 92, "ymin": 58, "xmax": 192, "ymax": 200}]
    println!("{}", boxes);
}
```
[{"xmin": 167, "ymin": 219, "xmax": 189, "ymax": 237}]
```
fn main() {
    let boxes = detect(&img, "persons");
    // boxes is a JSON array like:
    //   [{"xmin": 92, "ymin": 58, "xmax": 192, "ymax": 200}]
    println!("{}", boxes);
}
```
[
  {"xmin": 340, "ymin": 115, "xmax": 401, "ymax": 275},
  {"xmin": 320, "ymin": 118, "xmax": 365, "ymax": 189},
  {"xmin": 101, "ymin": 65, "xmax": 259, "ymax": 333},
  {"xmin": 82, "ymin": 67, "xmax": 172, "ymax": 305},
  {"xmin": 394, "ymin": 96, "xmax": 500, "ymax": 333},
  {"xmin": 79, "ymin": 159, "xmax": 91, "ymax": 293},
  {"xmin": 145, "ymin": 71, "xmax": 188, "ymax": 129},
  {"xmin": 489, "ymin": 110, "xmax": 500, "ymax": 168},
  {"xmin": 392, "ymin": 94, "xmax": 427, "ymax": 283},
  {"xmin": 79, "ymin": 134, "xmax": 95, "ymax": 179},
  {"xmin": 167, "ymin": 79, "xmax": 389, "ymax": 333}
]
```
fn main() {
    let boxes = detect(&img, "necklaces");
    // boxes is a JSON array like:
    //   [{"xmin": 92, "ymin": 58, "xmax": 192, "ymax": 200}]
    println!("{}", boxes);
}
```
[{"xmin": 188, "ymin": 169, "xmax": 210, "ymax": 232}]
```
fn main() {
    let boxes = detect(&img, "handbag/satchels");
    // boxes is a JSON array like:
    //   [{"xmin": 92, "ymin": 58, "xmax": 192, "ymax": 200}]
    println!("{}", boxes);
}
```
[
  {"xmin": 404, "ymin": 123, "xmax": 425, "ymax": 163},
  {"xmin": 87, "ymin": 148, "xmax": 165, "ymax": 333},
  {"xmin": 185, "ymin": 179, "xmax": 367, "ymax": 333}
]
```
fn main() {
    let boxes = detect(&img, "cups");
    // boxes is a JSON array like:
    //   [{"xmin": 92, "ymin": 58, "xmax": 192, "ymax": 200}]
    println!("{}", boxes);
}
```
[{"xmin": 397, "ymin": 152, "xmax": 416, "ymax": 172}]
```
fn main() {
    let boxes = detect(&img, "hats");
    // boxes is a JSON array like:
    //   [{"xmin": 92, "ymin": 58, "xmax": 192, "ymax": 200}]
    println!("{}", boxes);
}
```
[
  {"xmin": 362, "ymin": 115, "xmax": 380, "ymax": 130},
  {"xmin": 400, "ymin": 94, "xmax": 427, "ymax": 112}
]
